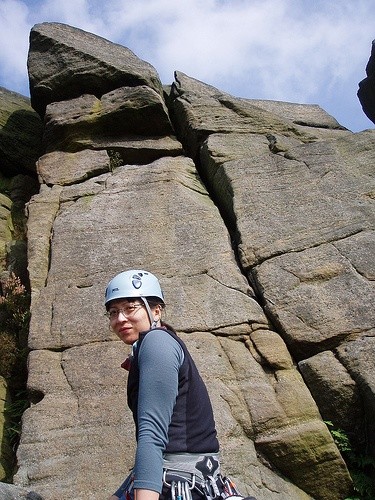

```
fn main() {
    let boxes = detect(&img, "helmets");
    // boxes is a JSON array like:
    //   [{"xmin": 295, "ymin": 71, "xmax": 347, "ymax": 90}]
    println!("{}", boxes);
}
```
[{"xmin": 105, "ymin": 269, "xmax": 164, "ymax": 305}]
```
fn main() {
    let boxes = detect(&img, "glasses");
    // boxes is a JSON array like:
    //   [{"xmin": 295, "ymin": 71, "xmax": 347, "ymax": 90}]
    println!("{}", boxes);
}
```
[{"xmin": 104, "ymin": 302, "xmax": 162, "ymax": 318}]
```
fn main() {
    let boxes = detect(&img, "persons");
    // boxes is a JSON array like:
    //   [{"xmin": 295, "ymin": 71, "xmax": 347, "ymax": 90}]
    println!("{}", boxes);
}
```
[{"xmin": 104, "ymin": 269, "xmax": 220, "ymax": 500}]
